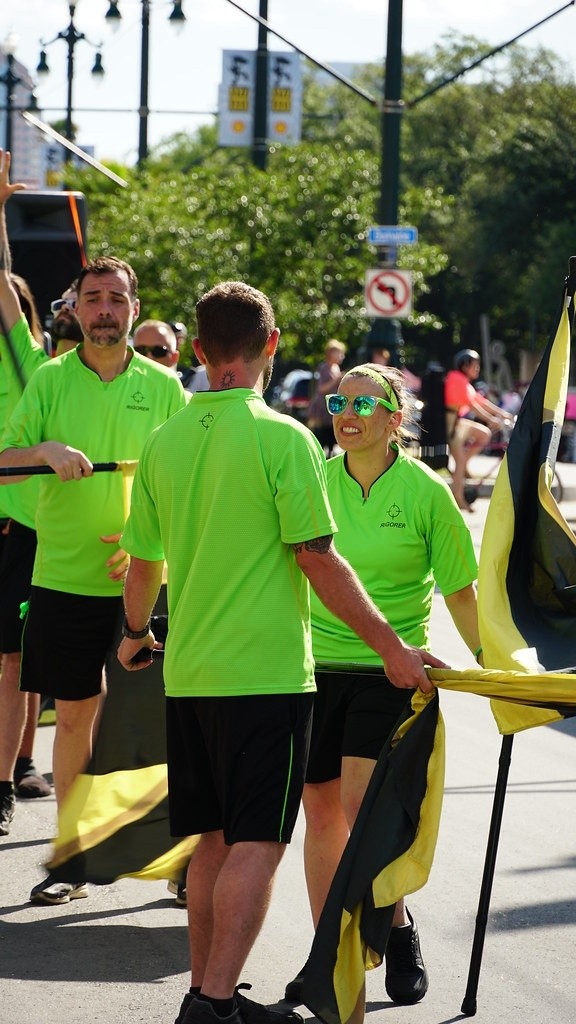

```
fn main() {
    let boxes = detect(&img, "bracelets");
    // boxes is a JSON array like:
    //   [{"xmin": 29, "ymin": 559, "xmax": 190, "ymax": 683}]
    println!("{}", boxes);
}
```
[
  {"xmin": 122, "ymin": 618, "xmax": 152, "ymax": 640},
  {"xmin": 474, "ymin": 646, "xmax": 483, "ymax": 664}
]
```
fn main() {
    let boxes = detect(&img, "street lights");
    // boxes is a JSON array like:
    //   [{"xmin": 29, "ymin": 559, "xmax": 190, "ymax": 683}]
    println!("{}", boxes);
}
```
[
  {"xmin": 104, "ymin": 0, "xmax": 187, "ymax": 173},
  {"xmin": 36, "ymin": 0, "xmax": 108, "ymax": 192}
]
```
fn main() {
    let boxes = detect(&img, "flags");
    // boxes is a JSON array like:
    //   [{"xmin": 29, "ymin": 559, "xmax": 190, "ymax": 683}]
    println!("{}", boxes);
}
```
[
  {"xmin": 44, "ymin": 454, "xmax": 203, "ymax": 882},
  {"xmin": 476, "ymin": 284, "xmax": 576, "ymax": 735}
]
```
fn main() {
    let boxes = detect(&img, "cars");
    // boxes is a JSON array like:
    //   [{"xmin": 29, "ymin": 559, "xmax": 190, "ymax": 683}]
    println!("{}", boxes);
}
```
[{"xmin": 268, "ymin": 367, "xmax": 317, "ymax": 424}]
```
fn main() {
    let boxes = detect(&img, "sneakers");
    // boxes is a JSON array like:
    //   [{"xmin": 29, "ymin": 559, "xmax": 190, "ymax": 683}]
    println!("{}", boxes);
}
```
[
  {"xmin": 174, "ymin": 983, "xmax": 304, "ymax": 1024},
  {"xmin": 30, "ymin": 873, "xmax": 90, "ymax": 904},
  {"xmin": 385, "ymin": 905, "xmax": 428, "ymax": 1002},
  {"xmin": 0, "ymin": 795, "xmax": 15, "ymax": 835},
  {"xmin": 13, "ymin": 759, "xmax": 51, "ymax": 798}
]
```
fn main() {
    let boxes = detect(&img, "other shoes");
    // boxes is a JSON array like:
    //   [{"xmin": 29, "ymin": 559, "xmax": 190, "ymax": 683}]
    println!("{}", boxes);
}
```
[
  {"xmin": 167, "ymin": 881, "xmax": 177, "ymax": 891},
  {"xmin": 177, "ymin": 887, "xmax": 187, "ymax": 905}
]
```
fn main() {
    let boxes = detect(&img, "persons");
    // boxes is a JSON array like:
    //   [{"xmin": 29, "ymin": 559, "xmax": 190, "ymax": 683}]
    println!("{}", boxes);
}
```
[
  {"xmin": 264, "ymin": 339, "xmax": 513, "ymax": 512},
  {"xmin": 285, "ymin": 362, "xmax": 479, "ymax": 1004},
  {"xmin": 0, "ymin": 146, "xmax": 192, "ymax": 905},
  {"xmin": 168, "ymin": 320, "xmax": 211, "ymax": 393},
  {"xmin": 117, "ymin": 280, "xmax": 451, "ymax": 1024}
]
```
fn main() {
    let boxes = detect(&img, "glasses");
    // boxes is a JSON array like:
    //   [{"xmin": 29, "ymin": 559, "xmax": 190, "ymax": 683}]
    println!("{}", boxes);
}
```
[
  {"xmin": 51, "ymin": 300, "xmax": 76, "ymax": 312},
  {"xmin": 134, "ymin": 344, "xmax": 169, "ymax": 358},
  {"xmin": 325, "ymin": 394, "xmax": 397, "ymax": 417}
]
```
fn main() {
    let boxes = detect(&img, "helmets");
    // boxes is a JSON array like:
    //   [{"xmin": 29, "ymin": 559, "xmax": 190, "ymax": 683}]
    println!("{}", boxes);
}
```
[{"xmin": 453, "ymin": 349, "xmax": 479, "ymax": 371}]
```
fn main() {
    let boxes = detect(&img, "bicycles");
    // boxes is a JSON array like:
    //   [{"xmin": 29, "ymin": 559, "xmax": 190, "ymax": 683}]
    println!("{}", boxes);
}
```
[{"xmin": 435, "ymin": 415, "xmax": 565, "ymax": 505}]
